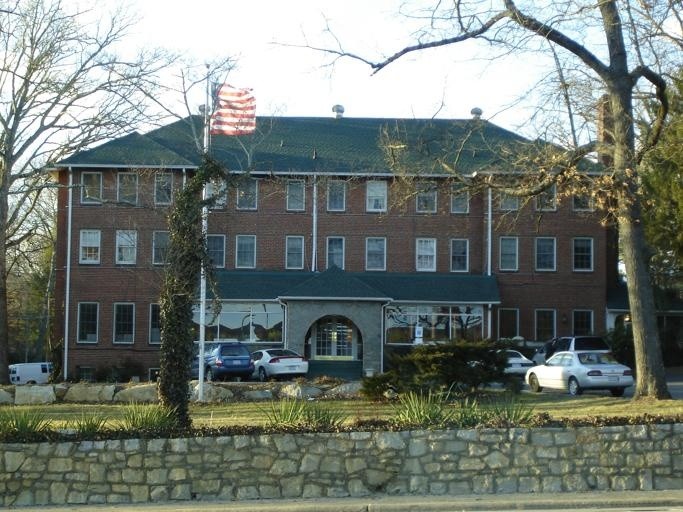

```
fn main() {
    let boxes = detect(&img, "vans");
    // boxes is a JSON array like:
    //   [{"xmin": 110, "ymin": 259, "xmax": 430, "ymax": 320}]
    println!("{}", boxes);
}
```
[
  {"xmin": 531, "ymin": 335, "xmax": 613, "ymax": 366},
  {"xmin": 5, "ymin": 362, "xmax": 54, "ymax": 385}
]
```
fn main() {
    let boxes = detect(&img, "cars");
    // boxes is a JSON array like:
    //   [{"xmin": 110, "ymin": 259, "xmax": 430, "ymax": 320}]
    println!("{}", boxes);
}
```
[
  {"xmin": 524, "ymin": 351, "xmax": 633, "ymax": 398},
  {"xmin": 467, "ymin": 350, "xmax": 534, "ymax": 376},
  {"xmin": 235, "ymin": 346, "xmax": 309, "ymax": 381}
]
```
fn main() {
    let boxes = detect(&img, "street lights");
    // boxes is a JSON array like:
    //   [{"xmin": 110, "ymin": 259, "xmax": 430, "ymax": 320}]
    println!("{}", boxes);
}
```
[{"xmin": 473, "ymin": 169, "xmax": 495, "ymax": 343}]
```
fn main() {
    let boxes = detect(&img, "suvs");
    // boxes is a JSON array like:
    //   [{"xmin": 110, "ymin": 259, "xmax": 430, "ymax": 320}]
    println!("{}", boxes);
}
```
[{"xmin": 190, "ymin": 341, "xmax": 253, "ymax": 384}]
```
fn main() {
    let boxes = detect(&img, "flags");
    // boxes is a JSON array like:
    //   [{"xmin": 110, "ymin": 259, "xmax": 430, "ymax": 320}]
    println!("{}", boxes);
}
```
[{"xmin": 207, "ymin": 80, "xmax": 256, "ymax": 136}]
